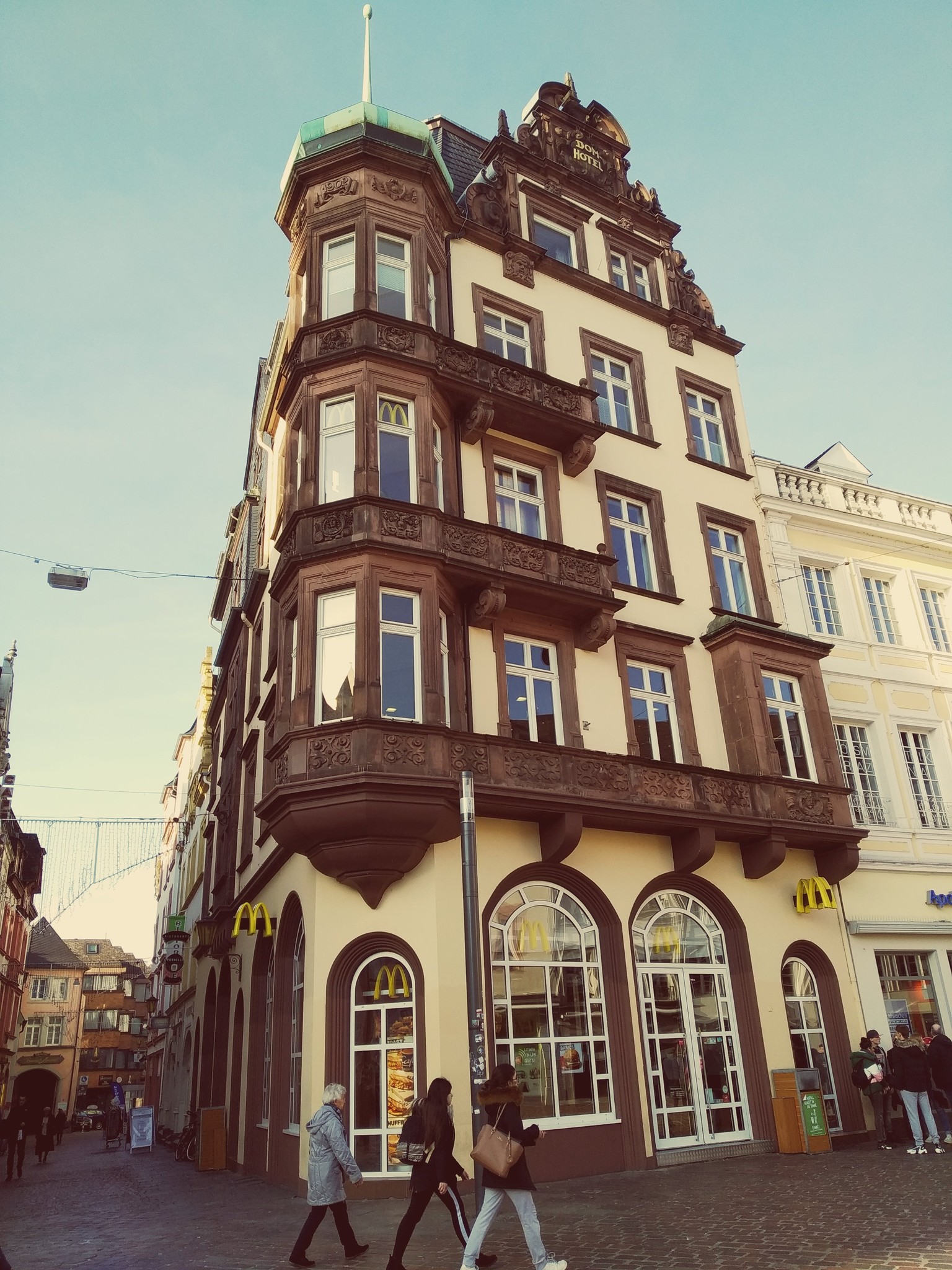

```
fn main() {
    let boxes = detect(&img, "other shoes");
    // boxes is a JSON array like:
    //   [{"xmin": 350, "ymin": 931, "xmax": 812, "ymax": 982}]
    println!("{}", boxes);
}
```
[
  {"xmin": 386, "ymin": 1254, "xmax": 407, "ymax": 1270},
  {"xmin": 475, "ymin": 1252, "xmax": 498, "ymax": 1267},
  {"xmin": 5, "ymin": 1169, "xmax": 22, "ymax": 1182},
  {"xmin": 56, "ymin": 1143, "xmax": 61, "ymax": 1146},
  {"xmin": 38, "ymin": 1159, "xmax": 46, "ymax": 1164}
]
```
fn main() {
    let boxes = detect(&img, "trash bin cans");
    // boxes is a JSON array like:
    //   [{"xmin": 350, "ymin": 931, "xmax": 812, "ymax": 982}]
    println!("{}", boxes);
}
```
[
  {"xmin": 771, "ymin": 1068, "xmax": 833, "ymax": 1155},
  {"xmin": 194, "ymin": 1105, "xmax": 227, "ymax": 1172}
]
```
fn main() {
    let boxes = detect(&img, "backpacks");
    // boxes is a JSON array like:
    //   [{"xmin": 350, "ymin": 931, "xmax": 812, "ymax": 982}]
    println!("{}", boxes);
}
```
[
  {"xmin": 396, "ymin": 1115, "xmax": 426, "ymax": 1165},
  {"xmin": 851, "ymin": 1058, "xmax": 873, "ymax": 1090}
]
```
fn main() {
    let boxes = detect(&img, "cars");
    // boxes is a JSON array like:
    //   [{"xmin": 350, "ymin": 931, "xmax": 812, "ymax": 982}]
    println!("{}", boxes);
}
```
[{"xmin": 75, "ymin": 1108, "xmax": 106, "ymax": 1131}]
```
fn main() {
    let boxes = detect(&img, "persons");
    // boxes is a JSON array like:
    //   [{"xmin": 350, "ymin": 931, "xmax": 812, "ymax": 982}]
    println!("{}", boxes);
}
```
[
  {"xmin": 33, "ymin": 1106, "xmax": 57, "ymax": 1164},
  {"xmin": 55, "ymin": 1108, "xmax": 66, "ymax": 1146},
  {"xmin": 283, "ymin": 1083, "xmax": 371, "ymax": 1267},
  {"xmin": 926, "ymin": 1024, "xmax": 952, "ymax": 1144},
  {"xmin": 3, "ymin": 1092, "xmax": 31, "ymax": 1183},
  {"xmin": 386, "ymin": 1076, "xmax": 501, "ymax": 1269},
  {"xmin": 888, "ymin": 1025, "xmax": 946, "ymax": 1155},
  {"xmin": 918, "ymin": 1037, "xmax": 952, "ymax": 1144},
  {"xmin": 457, "ymin": 1063, "xmax": 569, "ymax": 1270},
  {"xmin": 864, "ymin": 1030, "xmax": 905, "ymax": 1147},
  {"xmin": 792, "ymin": 1034, "xmax": 835, "ymax": 1120},
  {"xmin": 850, "ymin": 1036, "xmax": 893, "ymax": 1150}
]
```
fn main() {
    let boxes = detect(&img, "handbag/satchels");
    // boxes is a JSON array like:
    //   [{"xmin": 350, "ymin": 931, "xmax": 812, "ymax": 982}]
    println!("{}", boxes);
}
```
[{"xmin": 470, "ymin": 1102, "xmax": 525, "ymax": 1179}]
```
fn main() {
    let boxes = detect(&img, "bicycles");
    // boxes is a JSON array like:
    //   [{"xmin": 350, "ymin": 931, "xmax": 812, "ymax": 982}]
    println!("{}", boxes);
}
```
[{"xmin": 173, "ymin": 1110, "xmax": 199, "ymax": 1162}]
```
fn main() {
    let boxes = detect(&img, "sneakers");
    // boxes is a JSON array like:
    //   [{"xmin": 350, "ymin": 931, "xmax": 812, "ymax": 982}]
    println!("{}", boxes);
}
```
[
  {"xmin": 345, "ymin": 1244, "xmax": 369, "ymax": 1260},
  {"xmin": 944, "ymin": 1134, "xmax": 952, "ymax": 1144},
  {"xmin": 935, "ymin": 1145, "xmax": 946, "ymax": 1153},
  {"xmin": 877, "ymin": 1143, "xmax": 893, "ymax": 1150},
  {"xmin": 925, "ymin": 1135, "xmax": 933, "ymax": 1143},
  {"xmin": 906, "ymin": 1144, "xmax": 928, "ymax": 1155},
  {"xmin": 542, "ymin": 1260, "xmax": 567, "ymax": 1270},
  {"xmin": 289, "ymin": 1256, "xmax": 316, "ymax": 1268}
]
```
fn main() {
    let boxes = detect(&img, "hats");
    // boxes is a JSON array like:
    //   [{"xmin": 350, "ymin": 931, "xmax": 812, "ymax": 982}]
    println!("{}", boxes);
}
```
[
  {"xmin": 111, "ymin": 1097, "xmax": 120, "ymax": 1106},
  {"xmin": 866, "ymin": 1030, "xmax": 883, "ymax": 1039}
]
